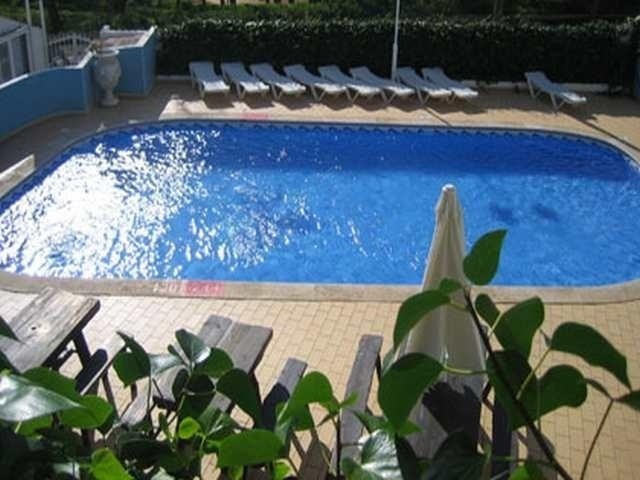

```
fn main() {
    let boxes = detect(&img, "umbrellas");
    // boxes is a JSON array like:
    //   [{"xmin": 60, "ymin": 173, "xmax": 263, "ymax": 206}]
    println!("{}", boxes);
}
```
[{"xmin": 383, "ymin": 183, "xmax": 490, "ymax": 452}]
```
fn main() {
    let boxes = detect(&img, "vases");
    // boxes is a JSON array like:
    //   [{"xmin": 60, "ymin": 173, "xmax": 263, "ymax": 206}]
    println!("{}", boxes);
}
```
[{"xmin": 92, "ymin": 47, "xmax": 123, "ymax": 108}]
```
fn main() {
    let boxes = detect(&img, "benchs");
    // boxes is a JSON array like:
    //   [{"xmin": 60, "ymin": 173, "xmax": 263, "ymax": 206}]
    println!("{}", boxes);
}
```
[
  {"xmin": 217, "ymin": 356, "xmax": 308, "ymax": 479},
  {"xmin": 327, "ymin": 330, "xmax": 384, "ymax": 479},
  {"xmin": 71, "ymin": 328, "xmax": 135, "ymax": 439}
]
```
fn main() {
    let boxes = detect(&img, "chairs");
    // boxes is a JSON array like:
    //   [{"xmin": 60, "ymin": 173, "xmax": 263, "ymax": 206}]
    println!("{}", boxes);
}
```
[
  {"xmin": 419, "ymin": 67, "xmax": 479, "ymax": 108},
  {"xmin": 248, "ymin": 62, "xmax": 307, "ymax": 102},
  {"xmin": 316, "ymin": 65, "xmax": 383, "ymax": 106},
  {"xmin": 221, "ymin": 63, "xmax": 270, "ymax": 102},
  {"xmin": 393, "ymin": 67, "xmax": 452, "ymax": 107},
  {"xmin": 281, "ymin": 62, "xmax": 347, "ymax": 103},
  {"xmin": 524, "ymin": 71, "xmax": 587, "ymax": 110},
  {"xmin": 189, "ymin": 62, "xmax": 232, "ymax": 103},
  {"xmin": 350, "ymin": 66, "xmax": 415, "ymax": 107}
]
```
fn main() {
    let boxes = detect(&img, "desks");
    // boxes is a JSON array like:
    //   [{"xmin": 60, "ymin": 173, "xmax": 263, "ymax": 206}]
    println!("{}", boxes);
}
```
[
  {"xmin": 0, "ymin": 285, "xmax": 103, "ymax": 374},
  {"xmin": 96, "ymin": 314, "xmax": 274, "ymax": 447}
]
